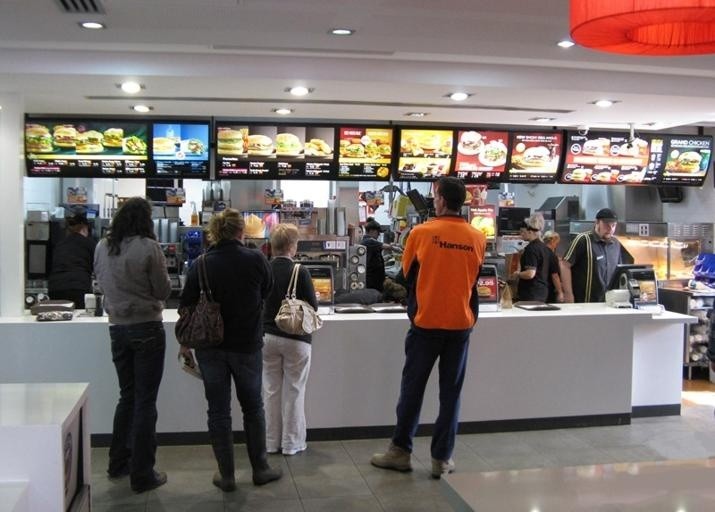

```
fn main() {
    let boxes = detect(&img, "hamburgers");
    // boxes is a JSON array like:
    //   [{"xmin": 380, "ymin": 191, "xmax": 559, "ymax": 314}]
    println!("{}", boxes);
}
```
[
  {"xmin": 477, "ymin": 287, "xmax": 492, "ymax": 299},
  {"xmin": 572, "ymin": 139, "xmax": 647, "ymax": 182},
  {"xmin": 679, "ymin": 151, "xmax": 701, "ymax": 172},
  {"xmin": 121, "ymin": 136, "xmax": 147, "ymax": 154},
  {"xmin": 461, "ymin": 131, "xmax": 507, "ymax": 162},
  {"xmin": 216, "ymin": 131, "xmax": 243, "ymax": 156},
  {"xmin": 339, "ymin": 140, "xmax": 391, "ymax": 157},
  {"xmin": 26, "ymin": 123, "xmax": 54, "ymax": 153},
  {"xmin": 277, "ymin": 133, "xmax": 300, "ymax": 157},
  {"xmin": 75, "ymin": 130, "xmax": 106, "ymax": 154},
  {"xmin": 248, "ymin": 135, "xmax": 274, "ymax": 156},
  {"xmin": 523, "ymin": 147, "xmax": 550, "ymax": 166},
  {"xmin": 53, "ymin": 126, "xmax": 79, "ymax": 148},
  {"xmin": 103, "ymin": 127, "xmax": 124, "ymax": 147}
]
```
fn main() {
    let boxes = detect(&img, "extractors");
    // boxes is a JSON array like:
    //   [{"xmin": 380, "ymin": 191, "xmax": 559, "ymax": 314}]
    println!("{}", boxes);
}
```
[{"xmin": 569, "ymin": 183, "xmax": 667, "ymax": 241}]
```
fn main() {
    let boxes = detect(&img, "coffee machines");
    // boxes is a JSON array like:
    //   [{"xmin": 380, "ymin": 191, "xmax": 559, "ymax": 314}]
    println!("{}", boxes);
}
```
[{"xmin": 295, "ymin": 236, "xmax": 349, "ymax": 295}]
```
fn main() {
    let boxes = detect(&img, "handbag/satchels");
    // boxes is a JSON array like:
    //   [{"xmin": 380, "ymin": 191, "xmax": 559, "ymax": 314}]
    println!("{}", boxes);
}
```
[
  {"xmin": 274, "ymin": 261, "xmax": 323, "ymax": 338},
  {"xmin": 175, "ymin": 254, "xmax": 225, "ymax": 348}
]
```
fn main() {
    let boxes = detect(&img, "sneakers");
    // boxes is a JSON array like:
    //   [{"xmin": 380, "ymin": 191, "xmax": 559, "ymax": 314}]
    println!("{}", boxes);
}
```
[
  {"xmin": 213, "ymin": 471, "xmax": 237, "ymax": 491},
  {"xmin": 431, "ymin": 454, "xmax": 457, "ymax": 480},
  {"xmin": 266, "ymin": 442, "xmax": 307, "ymax": 456},
  {"xmin": 251, "ymin": 467, "xmax": 283, "ymax": 485},
  {"xmin": 372, "ymin": 442, "xmax": 412, "ymax": 472},
  {"xmin": 108, "ymin": 467, "xmax": 130, "ymax": 479},
  {"xmin": 133, "ymin": 472, "xmax": 167, "ymax": 494}
]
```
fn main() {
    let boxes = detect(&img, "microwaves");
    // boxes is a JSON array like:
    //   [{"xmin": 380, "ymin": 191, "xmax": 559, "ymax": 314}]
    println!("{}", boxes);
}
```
[{"xmin": 498, "ymin": 207, "xmax": 531, "ymax": 235}]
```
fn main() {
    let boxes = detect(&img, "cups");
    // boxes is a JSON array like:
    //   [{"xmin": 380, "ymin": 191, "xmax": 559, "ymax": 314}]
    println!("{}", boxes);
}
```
[
  {"xmin": 74, "ymin": 178, "xmax": 81, "ymax": 186},
  {"xmin": 177, "ymin": 275, "xmax": 186, "ymax": 289},
  {"xmin": 427, "ymin": 158, "xmax": 448, "ymax": 177},
  {"xmin": 350, "ymin": 248, "xmax": 365, "ymax": 289},
  {"xmin": 202, "ymin": 180, "xmax": 230, "ymax": 201},
  {"xmin": 499, "ymin": 183, "xmax": 509, "ymax": 192},
  {"xmin": 242, "ymin": 214, "xmax": 278, "ymax": 238},
  {"xmin": 85, "ymin": 295, "xmax": 104, "ymax": 317},
  {"xmin": 547, "ymin": 144, "xmax": 559, "ymax": 158},
  {"xmin": 691, "ymin": 297, "xmax": 714, "ymax": 308}
]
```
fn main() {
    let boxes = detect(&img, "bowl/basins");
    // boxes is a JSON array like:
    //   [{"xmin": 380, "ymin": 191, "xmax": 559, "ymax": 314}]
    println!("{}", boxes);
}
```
[{"xmin": 281, "ymin": 199, "xmax": 313, "ymax": 210}]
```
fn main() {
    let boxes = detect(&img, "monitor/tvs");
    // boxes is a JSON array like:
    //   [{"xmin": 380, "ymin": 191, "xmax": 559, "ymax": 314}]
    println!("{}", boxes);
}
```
[
  {"xmin": 295, "ymin": 259, "xmax": 337, "ymax": 286},
  {"xmin": 606, "ymin": 263, "xmax": 654, "ymax": 292}
]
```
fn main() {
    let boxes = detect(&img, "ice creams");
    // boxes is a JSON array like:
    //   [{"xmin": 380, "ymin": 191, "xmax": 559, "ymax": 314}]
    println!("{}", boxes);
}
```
[{"xmin": 464, "ymin": 188, "xmax": 487, "ymax": 207}]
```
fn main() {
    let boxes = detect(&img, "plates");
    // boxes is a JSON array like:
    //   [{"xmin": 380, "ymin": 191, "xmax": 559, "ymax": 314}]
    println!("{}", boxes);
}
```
[
  {"xmin": 583, "ymin": 149, "xmax": 636, "ymax": 157},
  {"xmin": 398, "ymin": 167, "xmax": 421, "ymax": 175},
  {"xmin": 516, "ymin": 155, "xmax": 548, "ymax": 167},
  {"xmin": 26, "ymin": 140, "xmax": 124, "ymax": 154},
  {"xmin": 581, "ymin": 176, "xmax": 640, "ymax": 184},
  {"xmin": 153, "ymin": 151, "xmax": 202, "ymax": 156},
  {"xmin": 665, "ymin": 222, "xmax": 710, "ymax": 239},
  {"xmin": 457, "ymin": 140, "xmax": 506, "ymax": 166},
  {"xmin": 218, "ymin": 153, "xmax": 333, "ymax": 159},
  {"xmin": 343, "ymin": 153, "xmax": 391, "ymax": 158}
]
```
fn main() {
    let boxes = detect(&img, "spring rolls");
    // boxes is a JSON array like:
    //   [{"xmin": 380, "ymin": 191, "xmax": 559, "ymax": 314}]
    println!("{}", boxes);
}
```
[{"xmin": 178, "ymin": 138, "xmax": 203, "ymax": 154}]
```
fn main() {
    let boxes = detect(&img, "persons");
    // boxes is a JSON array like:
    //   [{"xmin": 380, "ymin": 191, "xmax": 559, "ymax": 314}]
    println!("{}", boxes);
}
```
[
  {"xmin": 179, "ymin": 207, "xmax": 282, "ymax": 492},
  {"xmin": 371, "ymin": 175, "xmax": 487, "ymax": 479},
  {"xmin": 539, "ymin": 230, "xmax": 561, "ymax": 252},
  {"xmin": 359, "ymin": 221, "xmax": 408, "ymax": 304},
  {"xmin": 508, "ymin": 215, "xmax": 563, "ymax": 305},
  {"xmin": 47, "ymin": 215, "xmax": 95, "ymax": 310},
  {"xmin": 260, "ymin": 222, "xmax": 318, "ymax": 455},
  {"xmin": 560, "ymin": 208, "xmax": 635, "ymax": 304},
  {"xmin": 93, "ymin": 196, "xmax": 171, "ymax": 494}
]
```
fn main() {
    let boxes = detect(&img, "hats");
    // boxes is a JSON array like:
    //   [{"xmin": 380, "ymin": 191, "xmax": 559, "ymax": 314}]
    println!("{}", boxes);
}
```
[
  {"xmin": 596, "ymin": 207, "xmax": 618, "ymax": 224},
  {"xmin": 364, "ymin": 224, "xmax": 388, "ymax": 233}
]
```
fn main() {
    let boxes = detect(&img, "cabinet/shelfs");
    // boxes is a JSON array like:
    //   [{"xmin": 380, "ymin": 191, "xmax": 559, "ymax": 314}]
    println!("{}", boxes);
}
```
[{"xmin": 658, "ymin": 286, "xmax": 715, "ymax": 381}]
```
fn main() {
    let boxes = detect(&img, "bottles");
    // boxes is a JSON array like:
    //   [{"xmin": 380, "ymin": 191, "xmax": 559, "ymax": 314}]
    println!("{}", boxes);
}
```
[
  {"xmin": 318, "ymin": 199, "xmax": 345, "ymax": 236},
  {"xmin": 26, "ymin": 293, "xmax": 47, "ymax": 304},
  {"xmin": 190, "ymin": 202, "xmax": 199, "ymax": 226},
  {"xmin": 688, "ymin": 280, "xmax": 696, "ymax": 290},
  {"xmin": 152, "ymin": 218, "xmax": 178, "ymax": 242}
]
